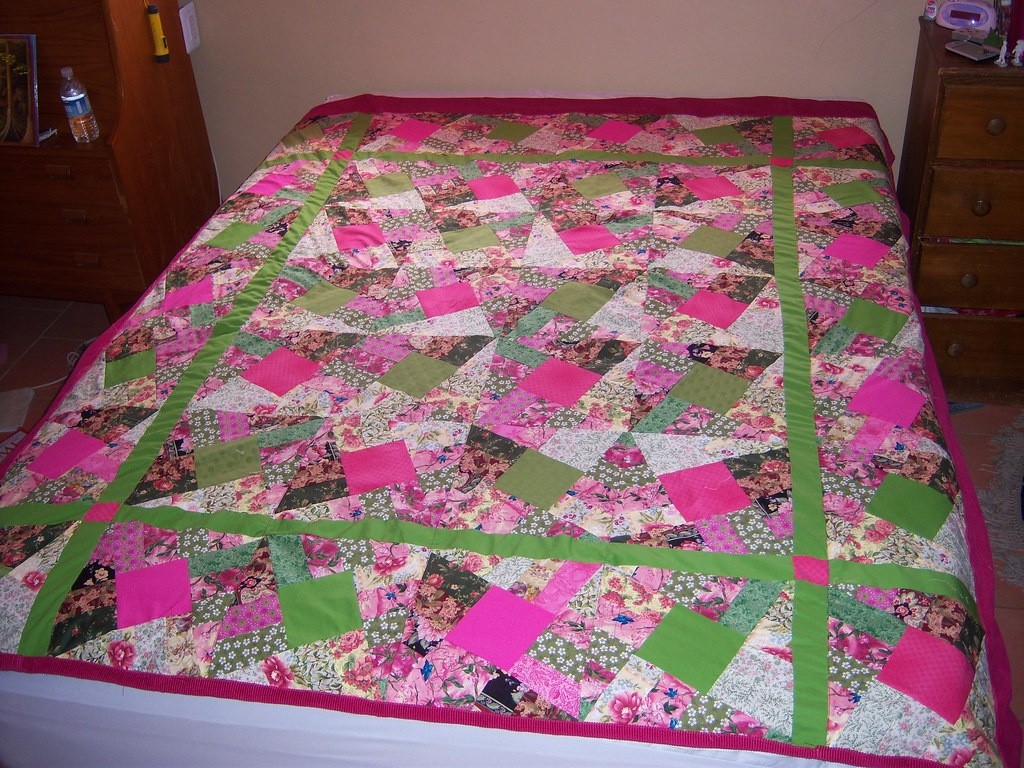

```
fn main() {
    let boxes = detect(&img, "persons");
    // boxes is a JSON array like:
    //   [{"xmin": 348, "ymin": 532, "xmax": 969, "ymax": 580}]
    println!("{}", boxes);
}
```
[
  {"xmin": 996, "ymin": 40, "xmax": 1008, "ymax": 64},
  {"xmin": 1012, "ymin": 39, "xmax": 1024, "ymax": 64}
]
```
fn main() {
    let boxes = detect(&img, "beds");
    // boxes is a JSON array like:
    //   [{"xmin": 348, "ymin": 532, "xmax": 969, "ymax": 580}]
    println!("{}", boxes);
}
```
[{"xmin": 0, "ymin": 92, "xmax": 1024, "ymax": 768}]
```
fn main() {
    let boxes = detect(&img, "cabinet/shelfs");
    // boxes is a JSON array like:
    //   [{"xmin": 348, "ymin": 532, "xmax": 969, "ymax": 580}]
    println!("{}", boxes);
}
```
[
  {"xmin": 896, "ymin": 15, "xmax": 1024, "ymax": 384},
  {"xmin": 0, "ymin": 0, "xmax": 220, "ymax": 327}
]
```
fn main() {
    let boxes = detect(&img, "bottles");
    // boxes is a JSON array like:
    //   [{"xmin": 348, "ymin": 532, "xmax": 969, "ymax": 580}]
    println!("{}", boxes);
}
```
[{"xmin": 59, "ymin": 67, "xmax": 99, "ymax": 143}]
